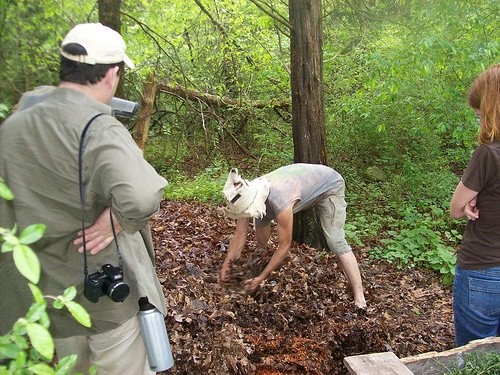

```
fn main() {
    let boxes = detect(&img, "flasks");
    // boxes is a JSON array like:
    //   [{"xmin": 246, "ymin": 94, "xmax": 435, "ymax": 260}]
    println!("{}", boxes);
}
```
[{"xmin": 136, "ymin": 296, "xmax": 174, "ymax": 372}]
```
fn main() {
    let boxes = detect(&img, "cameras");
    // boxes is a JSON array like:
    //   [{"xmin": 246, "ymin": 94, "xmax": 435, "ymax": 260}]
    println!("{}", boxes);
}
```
[{"xmin": 84, "ymin": 264, "xmax": 130, "ymax": 303}]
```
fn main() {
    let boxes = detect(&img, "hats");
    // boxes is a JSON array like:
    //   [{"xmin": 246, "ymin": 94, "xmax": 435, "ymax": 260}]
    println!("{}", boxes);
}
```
[
  {"xmin": 60, "ymin": 23, "xmax": 136, "ymax": 70},
  {"xmin": 224, "ymin": 167, "xmax": 270, "ymax": 218}
]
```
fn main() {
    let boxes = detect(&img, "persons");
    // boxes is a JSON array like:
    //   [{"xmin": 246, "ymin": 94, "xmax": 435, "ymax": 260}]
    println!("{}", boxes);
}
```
[
  {"xmin": 219, "ymin": 163, "xmax": 368, "ymax": 312},
  {"xmin": 0, "ymin": 22, "xmax": 168, "ymax": 375},
  {"xmin": 449, "ymin": 63, "xmax": 500, "ymax": 347}
]
